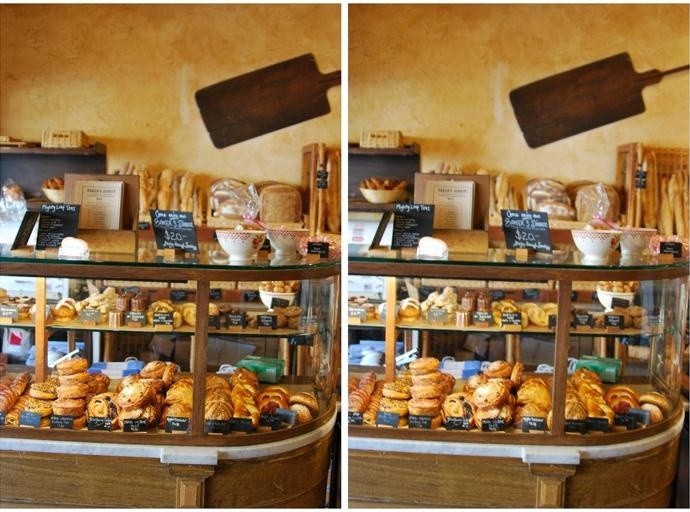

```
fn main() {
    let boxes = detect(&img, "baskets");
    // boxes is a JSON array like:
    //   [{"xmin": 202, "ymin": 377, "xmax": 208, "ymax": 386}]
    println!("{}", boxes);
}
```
[
  {"xmin": 40, "ymin": 186, "xmax": 67, "ymax": 203},
  {"xmin": 359, "ymin": 126, "xmax": 405, "ymax": 151},
  {"xmin": 40, "ymin": 126, "xmax": 90, "ymax": 150},
  {"xmin": 357, "ymin": 185, "xmax": 410, "ymax": 205}
]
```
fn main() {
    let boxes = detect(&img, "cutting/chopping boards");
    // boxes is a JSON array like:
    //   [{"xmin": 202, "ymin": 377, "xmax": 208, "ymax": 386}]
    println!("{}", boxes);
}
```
[
  {"xmin": 508, "ymin": 51, "xmax": 664, "ymax": 149},
  {"xmin": 193, "ymin": 52, "xmax": 341, "ymax": 150}
]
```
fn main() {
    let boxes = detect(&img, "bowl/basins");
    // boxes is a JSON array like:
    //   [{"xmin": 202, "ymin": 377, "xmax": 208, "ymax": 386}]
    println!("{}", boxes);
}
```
[
  {"xmin": 618, "ymin": 226, "xmax": 657, "ymax": 256},
  {"xmin": 359, "ymin": 187, "xmax": 404, "ymax": 204},
  {"xmin": 266, "ymin": 228, "xmax": 311, "ymax": 258},
  {"xmin": 215, "ymin": 229, "xmax": 268, "ymax": 264},
  {"xmin": 569, "ymin": 227, "xmax": 624, "ymax": 262},
  {"xmin": 595, "ymin": 286, "xmax": 638, "ymax": 315},
  {"xmin": 258, "ymin": 288, "xmax": 301, "ymax": 313},
  {"xmin": 41, "ymin": 188, "xmax": 64, "ymax": 203}
]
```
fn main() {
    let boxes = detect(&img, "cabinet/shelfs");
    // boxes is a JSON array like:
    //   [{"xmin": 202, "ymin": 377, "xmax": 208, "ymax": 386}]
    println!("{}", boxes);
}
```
[
  {"xmin": 0, "ymin": 141, "xmax": 108, "ymax": 216},
  {"xmin": 612, "ymin": 141, "xmax": 690, "ymax": 508},
  {"xmin": 295, "ymin": 142, "xmax": 343, "ymax": 507},
  {"xmin": 347, "ymin": 229, "xmax": 690, "ymax": 509},
  {"xmin": 348, "ymin": 141, "xmax": 422, "ymax": 215},
  {"xmin": 0, "ymin": 222, "xmax": 341, "ymax": 507}
]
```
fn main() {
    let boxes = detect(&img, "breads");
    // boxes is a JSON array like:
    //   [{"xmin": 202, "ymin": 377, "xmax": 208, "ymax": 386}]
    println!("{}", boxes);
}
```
[
  {"xmin": 360, "ymin": 150, "xmax": 690, "ymax": 237},
  {"xmin": 0, "ymin": 129, "xmax": 341, "ymax": 259},
  {"xmin": 348, "ymin": 357, "xmax": 670, "ymax": 430},
  {"xmin": 0, "ymin": 280, "xmax": 303, "ymax": 330},
  {"xmin": 348, "ymin": 280, "xmax": 649, "ymax": 331},
  {"xmin": 0, "ymin": 352, "xmax": 319, "ymax": 430}
]
